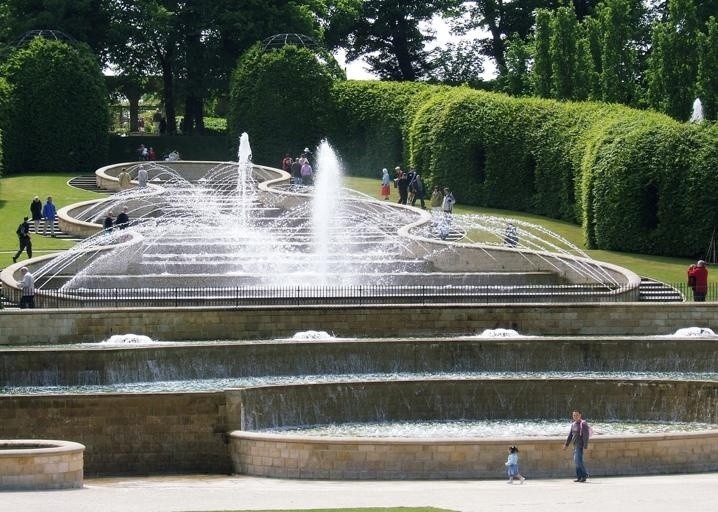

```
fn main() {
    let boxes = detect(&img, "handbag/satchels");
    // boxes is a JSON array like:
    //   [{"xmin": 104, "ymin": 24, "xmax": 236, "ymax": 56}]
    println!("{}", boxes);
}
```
[{"xmin": 16, "ymin": 224, "xmax": 26, "ymax": 237}]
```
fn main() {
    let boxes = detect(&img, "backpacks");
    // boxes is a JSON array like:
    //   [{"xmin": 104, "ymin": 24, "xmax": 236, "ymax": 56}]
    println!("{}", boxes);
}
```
[{"xmin": 573, "ymin": 420, "xmax": 593, "ymax": 437}]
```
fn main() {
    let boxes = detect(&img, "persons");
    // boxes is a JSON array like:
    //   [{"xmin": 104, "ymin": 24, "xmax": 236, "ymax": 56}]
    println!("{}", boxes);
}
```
[
  {"xmin": 115, "ymin": 168, "xmax": 132, "ymax": 191},
  {"xmin": 378, "ymin": 165, "xmax": 456, "ymax": 230},
  {"xmin": 125, "ymin": 107, "xmax": 166, "ymax": 163},
  {"xmin": 42, "ymin": 196, "xmax": 59, "ymax": 239},
  {"xmin": 117, "ymin": 205, "xmax": 131, "ymax": 231},
  {"xmin": 503, "ymin": 219, "xmax": 520, "ymax": 248},
  {"xmin": 29, "ymin": 195, "xmax": 43, "ymax": 234},
  {"xmin": 10, "ymin": 214, "xmax": 36, "ymax": 262},
  {"xmin": 686, "ymin": 260, "xmax": 711, "ymax": 302},
  {"xmin": 102, "ymin": 210, "xmax": 114, "ymax": 236},
  {"xmin": 560, "ymin": 409, "xmax": 593, "ymax": 483},
  {"xmin": 502, "ymin": 445, "xmax": 524, "ymax": 484},
  {"xmin": 137, "ymin": 164, "xmax": 150, "ymax": 190},
  {"xmin": 15, "ymin": 266, "xmax": 36, "ymax": 308},
  {"xmin": 281, "ymin": 146, "xmax": 316, "ymax": 192}
]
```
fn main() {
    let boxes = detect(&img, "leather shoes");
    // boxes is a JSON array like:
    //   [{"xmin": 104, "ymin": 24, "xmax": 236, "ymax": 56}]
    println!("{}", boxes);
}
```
[
  {"xmin": 575, "ymin": 476, "xmax": 582, "ymax": 482},
  {"xmin": 581, "ymin": 474, "xmax": 589, "ymax": 482}
]
